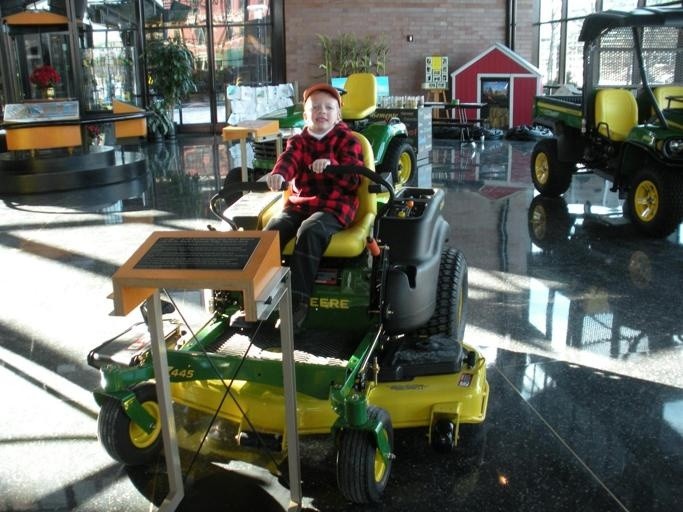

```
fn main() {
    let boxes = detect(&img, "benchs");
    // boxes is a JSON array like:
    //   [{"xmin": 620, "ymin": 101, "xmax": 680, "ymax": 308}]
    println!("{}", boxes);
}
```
[
  {"xmin": 0, "ymin": 110, "xmax": 156, "ymax": 160},
  {"xmin": 431, "ymin": 116, "xmax": 488, "ymax": 142},
  {"xmin": 431, "ymin": 122, "xmax": 472, "ymax": 144}
]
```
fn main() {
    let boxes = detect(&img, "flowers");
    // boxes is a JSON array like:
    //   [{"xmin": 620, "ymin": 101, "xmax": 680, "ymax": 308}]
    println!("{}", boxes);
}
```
[
  {"xmin": 27, "ymin": 64, "xmax": 61, "ymax": 92},
  {"xmin": 86, "ymin": 125, "xmax": 101, "ymax": 139}
]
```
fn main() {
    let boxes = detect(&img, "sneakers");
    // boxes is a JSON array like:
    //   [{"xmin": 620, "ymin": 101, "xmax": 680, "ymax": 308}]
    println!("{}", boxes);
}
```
[
  {"xmin": 228, "ymin": 293, "xmax": 265, "ymax": 331},
  {"xmin": 274, "ymin": 299, "xmax": 310, "ymax": 334}
]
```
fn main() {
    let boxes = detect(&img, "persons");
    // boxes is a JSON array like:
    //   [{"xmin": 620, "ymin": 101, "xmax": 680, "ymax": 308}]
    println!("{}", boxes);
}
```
[{"xmin": 229, "ymin": 83, "xmax": 363, "ymax": 331}]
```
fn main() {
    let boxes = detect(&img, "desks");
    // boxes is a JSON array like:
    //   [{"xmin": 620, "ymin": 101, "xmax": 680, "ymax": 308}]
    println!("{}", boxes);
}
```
[{"xmin": 423, "ymin": 101, "xmax": 488, "ymax": 143}]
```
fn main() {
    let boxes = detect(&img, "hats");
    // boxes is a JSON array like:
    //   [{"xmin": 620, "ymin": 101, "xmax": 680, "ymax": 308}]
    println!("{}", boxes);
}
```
[{"xmin": 301, "ymin": 82, "xmax": 344, "ymax": 111}]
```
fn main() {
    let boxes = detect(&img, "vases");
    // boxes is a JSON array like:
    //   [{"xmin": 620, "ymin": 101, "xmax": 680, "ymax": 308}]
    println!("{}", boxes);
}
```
[
  {"xmin": 90, "ymin": 136, "xmax": 100, "ymax": 145},
  {"xmin": 42, "ymin": 86, "xmax": 56, "ymax": 101}
]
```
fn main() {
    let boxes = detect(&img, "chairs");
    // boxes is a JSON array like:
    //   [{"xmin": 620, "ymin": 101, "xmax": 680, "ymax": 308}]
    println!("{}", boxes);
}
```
[
  {"xmin": 651, "ymin": 84, "xmax": 683, "ymax": 123},
  {"xmin": 593, "ymin": 85, "xmax": 640, "ymax": 144},
  {"xmin": 255, "ymin": 128, "xmax": 379, "ymax": 259},
  {"xmin": 332, "ymin": 70, "xmax": 381, "ymax": 121}
]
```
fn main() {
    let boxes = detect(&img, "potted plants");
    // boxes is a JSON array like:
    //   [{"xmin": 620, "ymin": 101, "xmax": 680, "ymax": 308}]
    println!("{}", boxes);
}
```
[
  {"xmin": 132, "ymin": 34, "xmax": 199, "ymax": 141},
  {"xmin": 143, "ymin": 96, "xmax": 172, "ymax": 144}
]
